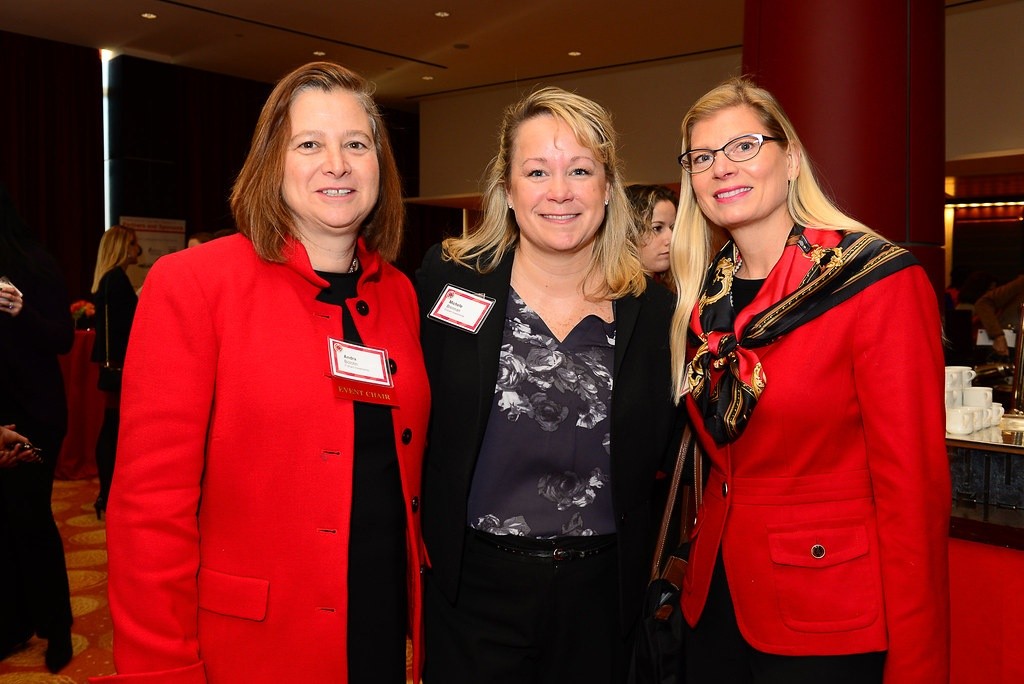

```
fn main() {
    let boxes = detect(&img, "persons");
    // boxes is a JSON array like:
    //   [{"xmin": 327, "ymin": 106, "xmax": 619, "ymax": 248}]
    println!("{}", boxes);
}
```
[
  {"xmin": 105, "ymin": 63, "xmax": 433, "ymax": 684},
  {"xmin": 943, "ymin": 220, "xmax": 1024, "ymax": 384},
  {"xmin": 0, "ymin": 178, "xmax": 75, "ymax": 671},
  {"xmin": 188, "ymin": 228, "xmax": 236, "ymax": 248},
  {"xmin": 668, "ymin": 78, "xmax": 953, "ymax": 684},
  {"xmin": 621, "ymin": 185, "xmax": 677, "ymax": 283},
  {"xmin": 91, "ymin": 226, "xmax": 138, "ymax": 519},
  {"xmin": 135, "ymin": 86, "xmax": 678, "ymax": 684}
]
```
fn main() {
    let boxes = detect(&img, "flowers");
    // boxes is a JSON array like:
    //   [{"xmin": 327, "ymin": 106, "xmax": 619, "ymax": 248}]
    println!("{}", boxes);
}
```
[{"xmin": 71, "ymin": 299, "xmax": 96, "ymax": 318}]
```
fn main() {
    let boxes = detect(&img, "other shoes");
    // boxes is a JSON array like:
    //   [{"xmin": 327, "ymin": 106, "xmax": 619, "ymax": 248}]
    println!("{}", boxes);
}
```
[
  {"xmin": 44, "ymin": 626, "xmax": 73, "ymax": 675},
  {"xmin": 93, "ymin": 497, "xmax": 107, "ymax": 521}
]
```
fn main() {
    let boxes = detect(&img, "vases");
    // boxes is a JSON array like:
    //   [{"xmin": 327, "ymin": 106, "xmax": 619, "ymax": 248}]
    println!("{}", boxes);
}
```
[{"xmin": 74, "ymin": 317, "xmax": 90, "ymax": 329}]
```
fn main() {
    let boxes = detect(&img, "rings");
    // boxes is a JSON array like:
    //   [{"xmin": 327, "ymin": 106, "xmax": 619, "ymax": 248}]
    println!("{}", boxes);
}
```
[{"xmin": 10, "ymin": 302, "xmax": 14, "ymax": 308}]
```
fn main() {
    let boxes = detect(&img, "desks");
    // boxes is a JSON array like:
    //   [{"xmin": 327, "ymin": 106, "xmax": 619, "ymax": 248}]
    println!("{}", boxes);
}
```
[{"xmin": 948, "ymin": 415, "xmax": 1024, "ymax": 551}]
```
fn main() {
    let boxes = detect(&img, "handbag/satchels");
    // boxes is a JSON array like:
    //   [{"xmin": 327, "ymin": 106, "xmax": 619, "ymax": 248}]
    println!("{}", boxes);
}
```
[{"xmin": 629, "ymin": 424, "xmax": 707, "ymax": 684}]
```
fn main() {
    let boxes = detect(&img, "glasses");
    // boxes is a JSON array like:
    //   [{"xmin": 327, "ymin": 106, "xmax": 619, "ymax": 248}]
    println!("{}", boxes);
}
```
[{"xmin": 678, "ymin": 133, "xmax": 787, "ymax": 174}]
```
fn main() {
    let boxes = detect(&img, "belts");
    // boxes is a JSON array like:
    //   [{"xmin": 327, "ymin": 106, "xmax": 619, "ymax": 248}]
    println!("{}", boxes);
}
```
[{"xmin": 474, "ymin": 530, "xmax": 620, "ymax": 561}]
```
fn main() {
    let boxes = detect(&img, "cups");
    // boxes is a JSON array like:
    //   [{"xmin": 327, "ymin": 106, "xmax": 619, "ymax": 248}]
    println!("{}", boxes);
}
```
[{"xmin": 945, "ymin": 366, "xmax": 1004, "ymax": 435}]
